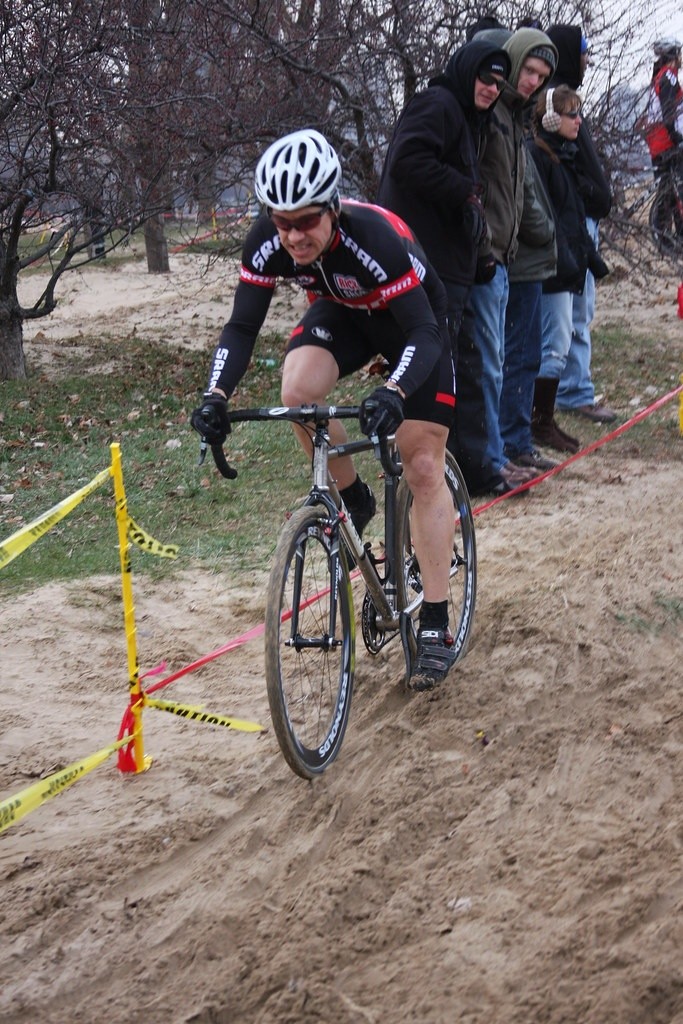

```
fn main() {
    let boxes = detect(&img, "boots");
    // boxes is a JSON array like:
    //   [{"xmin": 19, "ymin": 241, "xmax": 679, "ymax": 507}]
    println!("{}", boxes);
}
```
[{"xmin": 531, "ymin": 377, "xmax": 579, "ymax": 454}]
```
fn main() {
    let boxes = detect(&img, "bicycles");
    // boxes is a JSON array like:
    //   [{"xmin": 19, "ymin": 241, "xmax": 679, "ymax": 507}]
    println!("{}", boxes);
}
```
[{"xmin": 197, "ymin": 400, "xmax": 479, "ymax": 780}]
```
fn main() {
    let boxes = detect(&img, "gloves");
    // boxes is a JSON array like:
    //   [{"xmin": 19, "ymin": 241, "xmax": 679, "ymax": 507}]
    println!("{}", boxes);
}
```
[
  {"xmin": 190, "ymin": 391, "xmax": 232, "ymax": 444},
  {"xmin": 359, "ymin": 386, "xmax": 405, "ymax": 436},
  {"xmin": 476, "ymin": 252, "xmax": 497, "ymax": 280}
]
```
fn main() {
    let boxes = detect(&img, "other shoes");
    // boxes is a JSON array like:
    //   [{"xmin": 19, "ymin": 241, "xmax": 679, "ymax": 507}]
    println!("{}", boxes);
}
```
[
  {"xmin": 494, "ymin": 467, "xmax": 532, "ymax": 482},
  {"xmin": 514, "ymin": 449, "xmax": 563, "ymax": 469},
  {"xmin": 503, "ymin": 461, "xmax": 540, "ymax": 478},
  {"xmin": 471, "ymin": 480, "xmax": 529, "ymax": 499},
  {"xmin": 561, "ymin": 404, "xmax": 617, "ymax": 425}
]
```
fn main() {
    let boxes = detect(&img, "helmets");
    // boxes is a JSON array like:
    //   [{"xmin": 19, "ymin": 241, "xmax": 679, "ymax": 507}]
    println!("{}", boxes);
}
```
[
  {"xmin": 254, "ymin": 129, "xmax": 342, "ymax": 217},
  {"xmin": 652, "ymin": 36, "xmax": 683, "ymax": 59}
]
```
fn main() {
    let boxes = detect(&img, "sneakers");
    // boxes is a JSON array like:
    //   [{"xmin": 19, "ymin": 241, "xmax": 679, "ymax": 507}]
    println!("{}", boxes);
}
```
[
  {"xmin": 336, "ymin": 484, "xmax": 376, "ymax": 570},
  {"xmin": 411, "ymin": 627, "xmax": 456, "ymax": 691}
]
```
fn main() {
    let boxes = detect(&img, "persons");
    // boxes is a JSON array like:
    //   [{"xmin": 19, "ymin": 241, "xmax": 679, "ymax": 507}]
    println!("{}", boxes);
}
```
[
  {"xmin": 376, "ymin": 41, "xmax": 511, "ymax": 497},
  {"xmin": 639, "ymin": 37, "xmax": 683, "ymax": 246},
  {"xmin": 457, "ymin": 15, "xmax": 560, "ymax": 487},
  {"xmin": 525, "ymin": 83, "xmax": 609, "ymax": 452},
  {"xmin": 545, "ymin": 21, "xmax": 618, "ymax": 424},
  {"xmin": 196, "ymin": 129, "xmax": 459, "ymax": 691}
]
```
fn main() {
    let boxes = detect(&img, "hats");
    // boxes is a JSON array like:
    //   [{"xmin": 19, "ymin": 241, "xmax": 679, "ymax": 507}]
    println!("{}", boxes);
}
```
[
  {"xmin": 579, "ymin": 36, "xmax": 588, "ymax": 54},
  {"xmin": 477, "ymin": 52, "xmax": 508, "ymax": 78},
  {"xmin": 531, "ymin": 45, "xmax": 556, "ymax": 75}
]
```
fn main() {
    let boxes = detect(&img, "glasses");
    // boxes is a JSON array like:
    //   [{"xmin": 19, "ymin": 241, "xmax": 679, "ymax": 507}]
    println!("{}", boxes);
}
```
[
  {"xmin": 581, "ymin": 49, "xmax": 588, "ymax": 55},
  {"xmin": 266, "ymin": 189, "xmax": 338, "ymax": 232},
  {"xmin": 476, "ymin": 71, "xmax": 507, "ymax": 91},
  {"xmin": 563, "ymin": 109, "xmax": 583, "ymax": 119}
]
